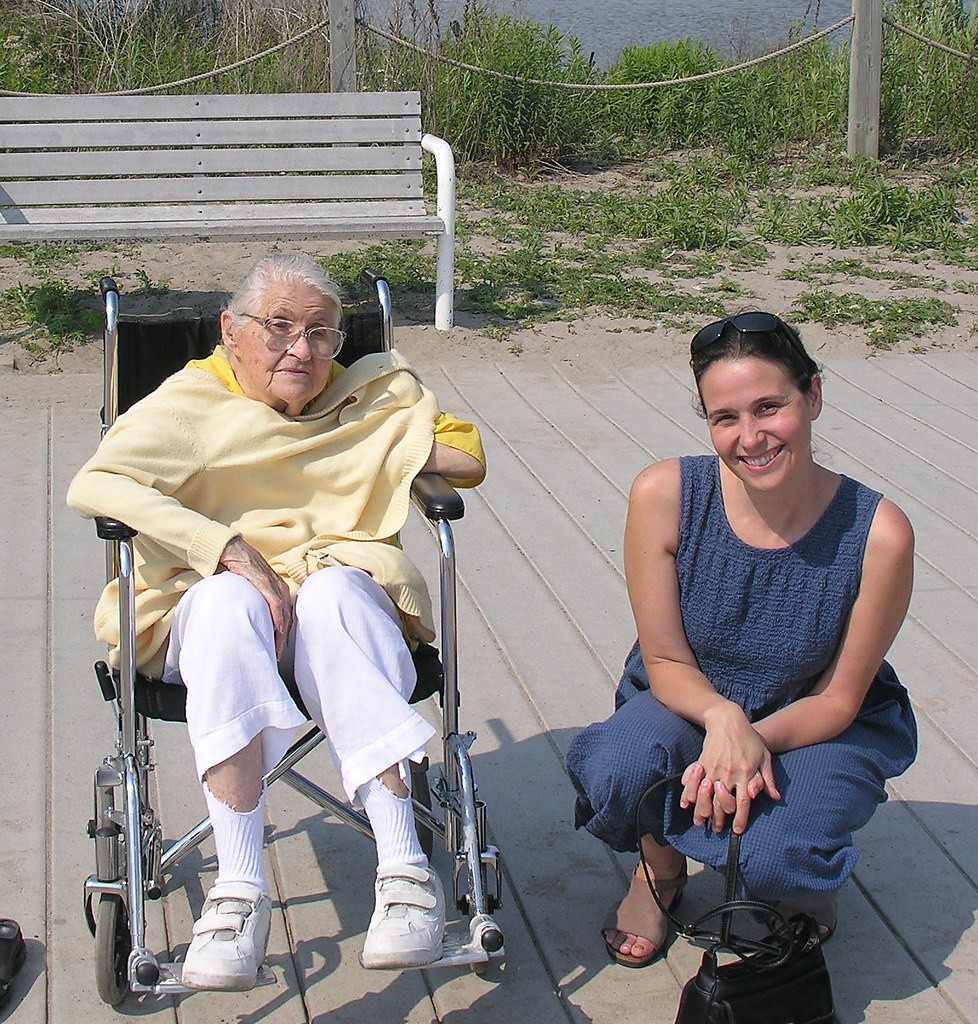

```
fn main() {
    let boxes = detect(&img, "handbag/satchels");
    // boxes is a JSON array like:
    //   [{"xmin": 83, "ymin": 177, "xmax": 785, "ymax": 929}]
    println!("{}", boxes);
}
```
[{"xmin": 673, "ymin": 901, "xmax": 835, "ymax": 1024}]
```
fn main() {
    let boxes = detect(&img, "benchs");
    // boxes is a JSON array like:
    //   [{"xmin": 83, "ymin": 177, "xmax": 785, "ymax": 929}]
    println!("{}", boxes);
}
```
[{"xmin": 0, "ymin": 89, "xmax": 456, "ymax": 332}]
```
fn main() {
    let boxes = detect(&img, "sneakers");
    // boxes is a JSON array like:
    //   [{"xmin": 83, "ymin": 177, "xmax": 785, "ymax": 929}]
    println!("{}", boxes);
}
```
[
  {"xmin": 362, "ymin": 864, "xmax": 444, "ymax": 969},
  {"xmin": 181, "ymin": 885, "xmax": 272, "ymax": 991}
]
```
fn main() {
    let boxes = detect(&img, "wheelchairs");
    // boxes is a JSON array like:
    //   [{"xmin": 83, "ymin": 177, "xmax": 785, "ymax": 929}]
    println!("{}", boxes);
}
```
[{"xmin": 79, "ymin": 263, "xmax": 510, "ymax": 1012}]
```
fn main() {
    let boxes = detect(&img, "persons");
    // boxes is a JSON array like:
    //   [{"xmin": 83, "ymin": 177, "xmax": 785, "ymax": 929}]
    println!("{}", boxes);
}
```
[
  {"xmin": 566, "ymin": 311, "xmax": 918, "ymax": 969},
  {"xmin": 66, "ymin": 253, "xmax": 487, "ymax": 990}
]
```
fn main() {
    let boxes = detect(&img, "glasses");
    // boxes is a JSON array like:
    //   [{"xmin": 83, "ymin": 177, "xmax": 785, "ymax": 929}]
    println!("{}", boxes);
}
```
[
  {"xmin": 689, "ymin": 313, "xmax": 811, "ymax": 415},
  {"xmin": 233, "ymin": 311, "xmax": 347, "ymax": 361}
]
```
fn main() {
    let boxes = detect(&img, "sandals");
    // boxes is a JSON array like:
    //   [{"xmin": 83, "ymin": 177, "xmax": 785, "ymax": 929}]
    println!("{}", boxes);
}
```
[
  {"xmin": 600, "ymin": 853, "xmax": 688, "ymax": 969},
  {"xmin": 770, "ymin": 884, "xmax": 837, "ymax": 944}
]
